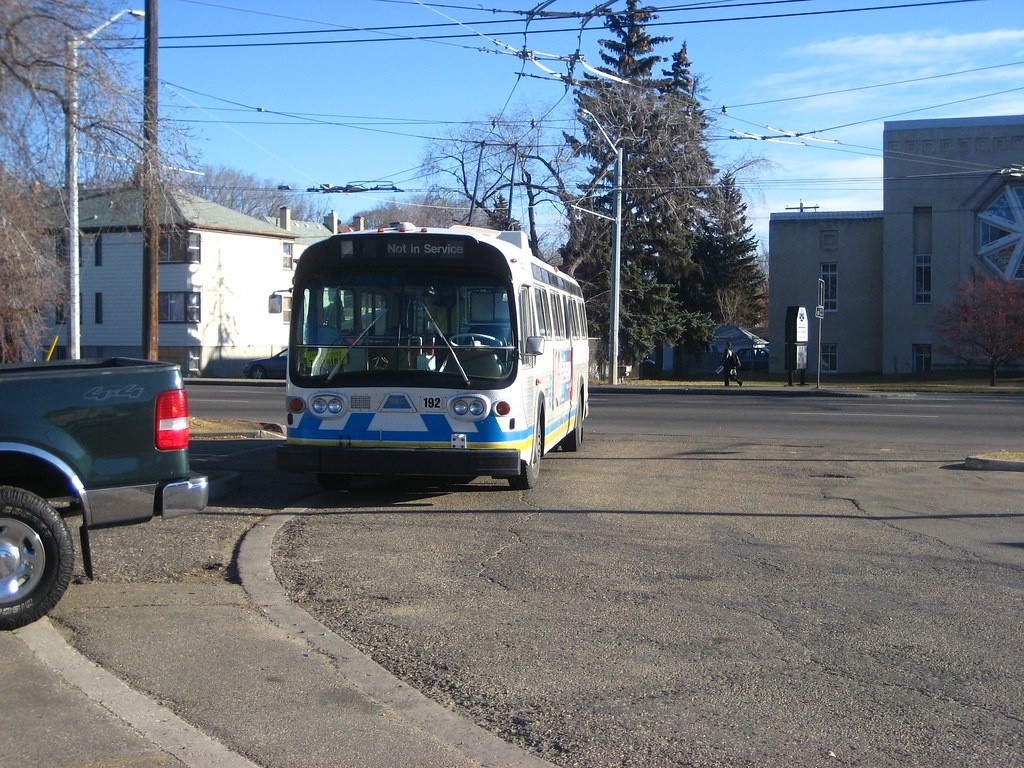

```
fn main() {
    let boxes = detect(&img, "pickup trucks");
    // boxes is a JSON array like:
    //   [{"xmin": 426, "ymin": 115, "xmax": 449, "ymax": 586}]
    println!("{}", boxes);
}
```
[{"xmin": 0, "ymin": 356, "xmax": 210, "ymax": 630}]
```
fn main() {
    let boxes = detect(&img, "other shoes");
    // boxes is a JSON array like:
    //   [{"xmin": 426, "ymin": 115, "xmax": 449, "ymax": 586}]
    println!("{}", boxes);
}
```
[{"xmin": 739, "ymin": 381, "xmax": 743, "ymax": 386}]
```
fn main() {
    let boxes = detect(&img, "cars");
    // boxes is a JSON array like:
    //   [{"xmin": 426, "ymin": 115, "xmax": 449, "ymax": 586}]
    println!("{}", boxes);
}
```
[
  {"xmin": 735, "ymin": 348, "xmax": 769, "ymax": 371},
  {"xmin": 242, "ymin": 347, "xmax": 287, "ymax": 379}
]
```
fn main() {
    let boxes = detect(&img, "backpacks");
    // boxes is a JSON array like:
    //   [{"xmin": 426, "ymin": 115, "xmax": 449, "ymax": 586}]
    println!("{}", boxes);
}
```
[{"xmin": 728, "ymin": 348, "xmax": 741, "ymax": 369}]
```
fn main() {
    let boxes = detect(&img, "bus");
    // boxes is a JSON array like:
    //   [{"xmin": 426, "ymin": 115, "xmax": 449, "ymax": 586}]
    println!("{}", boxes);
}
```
[{"xmin": 284, "ymin": 221, "xmax": 590, "ymax": 490}]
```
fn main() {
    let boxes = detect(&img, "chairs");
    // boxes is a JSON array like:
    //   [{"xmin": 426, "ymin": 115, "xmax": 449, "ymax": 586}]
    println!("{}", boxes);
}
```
[{"xmin": 337, "ymin": 323, "xmax": 513, "ymax": 367}]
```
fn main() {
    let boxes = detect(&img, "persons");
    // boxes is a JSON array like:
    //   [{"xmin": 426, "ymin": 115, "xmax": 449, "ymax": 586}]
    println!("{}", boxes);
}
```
[{"xmin": 716, "ymin": 341, "xmax": 743, "ymax": 386}]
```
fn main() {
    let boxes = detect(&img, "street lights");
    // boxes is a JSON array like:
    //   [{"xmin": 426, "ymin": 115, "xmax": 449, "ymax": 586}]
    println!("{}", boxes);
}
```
[
  {"xmin": 577, "ymin": 108, "xmax": 624, "ymax": 385},
  {"xmin": 64, "ymin": 8, "xmax": 146, "ymax": 359}
]
